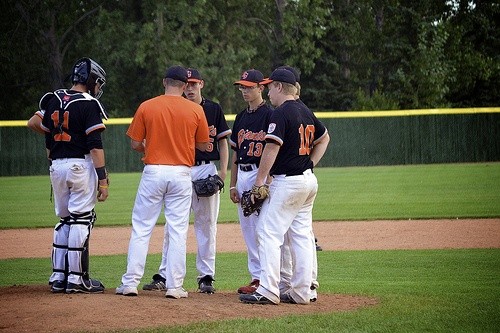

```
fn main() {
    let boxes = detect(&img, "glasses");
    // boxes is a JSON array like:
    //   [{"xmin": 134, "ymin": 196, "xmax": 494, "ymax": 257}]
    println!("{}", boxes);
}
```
[{"xmin": 238, "ymin": 86, "xmax": 258, "ymax": 91}]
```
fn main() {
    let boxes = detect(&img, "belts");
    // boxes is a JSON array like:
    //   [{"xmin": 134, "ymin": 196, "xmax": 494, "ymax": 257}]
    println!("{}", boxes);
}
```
[
  {"xmin": 285, "ymin": 171, "xmax": 302, "ymax": 177},
  {"xmin": 67, "ymin": 154, "xmax": 84, "ymax": 158},
  {"xmin": 195, "ymin": 160, "xmax": 211, "ymax": 167},
  {"xmin": 240, "ymin": 164, "xmax": 254, "ymax": 171}
]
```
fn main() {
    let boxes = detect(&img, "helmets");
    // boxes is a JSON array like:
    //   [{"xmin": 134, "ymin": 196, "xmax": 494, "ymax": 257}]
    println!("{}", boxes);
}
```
[{"xmin": 72, "ymin": 61, "xmax": 89, "ymax": 83}]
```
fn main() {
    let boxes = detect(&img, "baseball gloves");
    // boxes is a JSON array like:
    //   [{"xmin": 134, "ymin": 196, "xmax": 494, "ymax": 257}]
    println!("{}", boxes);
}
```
[
  {"xmin": 192, "ymin": 174, "xmax": 224, "ymax": 197},
  {"xmin": 241, "ymin": 185, "xmax": 269, "ymax": 217}
]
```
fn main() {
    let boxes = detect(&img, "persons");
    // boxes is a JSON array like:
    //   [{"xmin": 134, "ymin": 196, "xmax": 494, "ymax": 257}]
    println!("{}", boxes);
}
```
[
  {"xmin": 142, "ymin": 66, "xmax": 232, "ymax": 294},
  {"xmin": 115, "ymin": 66, "xmax": 208, "ymax": 299},
  {"xmin": 230, "ymin": 69, "xmax": 295, "ymax": 296},
  {"xmin": 28, "ymin": 58, "xmax": 110, "ymax": 293},
  {"xmin": 239, "ymin": 64, "xmax": 332, "ymax": 306}
]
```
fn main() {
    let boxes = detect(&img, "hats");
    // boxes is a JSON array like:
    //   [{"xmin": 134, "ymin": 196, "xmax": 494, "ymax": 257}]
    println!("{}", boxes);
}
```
[
  {"xmin": 164, "ymin": 65, "xmax": 188, "ymax": 84},
  {"xmin": 277, "ymin": 66, "xmax": 300, "ymax": 82},
  {"xmin": 259, "ymin": 68, "xmax": 296, "ymax": 86},
  {"xmin": 233, "ymin": 69, "xmax": 264, "ymax": 87},
  {"xmin": 186, "ymin": 68, "xmax": 201, "ymax": 83}
]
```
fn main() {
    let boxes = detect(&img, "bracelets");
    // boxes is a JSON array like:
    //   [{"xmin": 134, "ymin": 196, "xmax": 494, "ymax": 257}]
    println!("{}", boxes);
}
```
[
  {"xmin": 95, "ymin": 166, "xmax": 107, "ymax": 180},
  {"xmin": 229, "ymin": 186, "xmax": 236, "ymax": 191},
  {"xmin": 99, "ymin": 185, "xmax": 108, "ymax": 189}
]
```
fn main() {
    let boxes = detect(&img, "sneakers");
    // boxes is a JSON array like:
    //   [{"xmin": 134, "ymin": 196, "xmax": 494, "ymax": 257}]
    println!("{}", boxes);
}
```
[
  {"xmin": 53, "ymin": 280, "xmax": 64, "ymax": 291},
  {"xmin": 115, "ymin": 283, "xmax": 138, "ymax": 296},
  {"xmin": 66, "ymin": 281, "xmax": 103, "ymax": 294},
  {"xmin": 239, "ymin": 292, "xmax": 273, "ymax": 305},
  {"xmin": 238, "ymin": 278, "xmax": 260, "ymax": 293},
  {"xmin": 167, "ymin": 284, "xmax": 188, "ymax": 300},
  {"xmin": 197, "ymin": 275, "xmax": 215, "ymax": 294},
  {"xmin": 143, "ymin": 273, "xmax": 166, "ymax": 291},
  {"xmin": 279, "ymin": 293, "xmax": 296, "ymax": 303}
]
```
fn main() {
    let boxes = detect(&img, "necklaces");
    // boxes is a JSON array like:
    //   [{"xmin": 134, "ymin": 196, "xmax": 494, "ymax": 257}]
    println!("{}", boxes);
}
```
[{"xmin": 247, "ymin": 100, "xmax": 264, "ymax": 112}]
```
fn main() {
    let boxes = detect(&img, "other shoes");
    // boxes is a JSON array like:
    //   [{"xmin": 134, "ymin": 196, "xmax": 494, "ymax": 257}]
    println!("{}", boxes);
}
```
[{"xmin": 315, "ymin": 244, "xmax": 322, "ymax": 251}]
